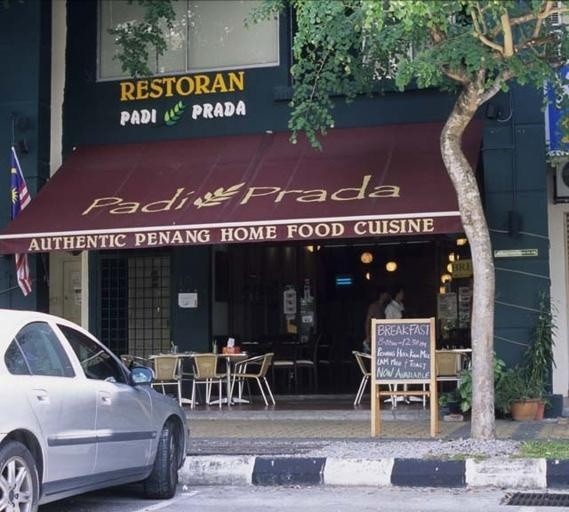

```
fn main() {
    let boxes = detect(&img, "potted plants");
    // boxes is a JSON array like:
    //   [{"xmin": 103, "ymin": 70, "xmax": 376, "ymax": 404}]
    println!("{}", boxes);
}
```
[{"xmin": 495, "ymin": 284, "xmax": 560, "ymax": 421}]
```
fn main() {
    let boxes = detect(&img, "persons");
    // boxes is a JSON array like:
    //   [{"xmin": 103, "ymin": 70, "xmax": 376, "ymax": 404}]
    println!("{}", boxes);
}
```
[
  {"xmin": 363, "ymin": 288, "xmax": 386, "ymax": 357},
  {"xmin": 384, "ymin": 287, "xmax": 406, "ymax": 320}
]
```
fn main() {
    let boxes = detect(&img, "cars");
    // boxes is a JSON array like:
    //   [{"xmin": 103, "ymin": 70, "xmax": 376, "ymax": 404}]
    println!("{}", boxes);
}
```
[{"xmin": 0, "ymin": 305, "xmax": 192, "ymax": 511}]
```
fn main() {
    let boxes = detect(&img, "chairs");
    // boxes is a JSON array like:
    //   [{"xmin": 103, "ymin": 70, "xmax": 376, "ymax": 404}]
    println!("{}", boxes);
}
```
[
  {"xmin": 351, "ymin": 347, "xmax": 472, "ymax": 411},
  {"xmin": 122, "ymin": 334, "xmax": 337, "ymax": 413}
]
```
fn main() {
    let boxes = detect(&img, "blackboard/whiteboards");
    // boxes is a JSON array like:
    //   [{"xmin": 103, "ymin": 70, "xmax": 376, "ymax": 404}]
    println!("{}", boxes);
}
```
[{"xmin": 370, "ymin": 317, "xmax": 436, "ymax": 384}]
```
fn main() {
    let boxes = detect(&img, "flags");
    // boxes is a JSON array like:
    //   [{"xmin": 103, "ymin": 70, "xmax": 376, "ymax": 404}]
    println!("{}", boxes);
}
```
[{"xmin": 9, "ymin": 146, "xmax": 33, "ymax": 297}]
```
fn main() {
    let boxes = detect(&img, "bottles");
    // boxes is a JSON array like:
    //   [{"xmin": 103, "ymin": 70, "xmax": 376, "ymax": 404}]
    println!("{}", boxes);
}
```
[
  {"xmin": 300, "ymin": 277, "xmax": 314, "ymax": 327},
  {"xmin": 213, "ymin": 339, "xmax": 218, "ymax": 353}
]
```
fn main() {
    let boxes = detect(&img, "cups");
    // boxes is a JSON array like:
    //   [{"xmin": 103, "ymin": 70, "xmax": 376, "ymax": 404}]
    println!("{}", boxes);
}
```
[{"xmin": 170, "ymin": 345, "xmax": 178, "ymax": 355}]
